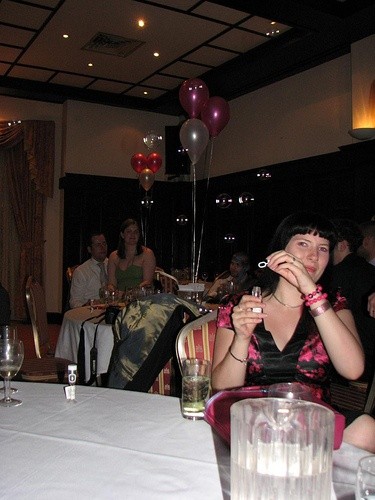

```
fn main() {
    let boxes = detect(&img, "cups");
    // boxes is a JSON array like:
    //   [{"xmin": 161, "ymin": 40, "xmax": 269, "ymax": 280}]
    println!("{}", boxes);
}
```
[
  {"xmin": 355, "ymin": 455, "xmax": 375, "ymax": 500},
  {"xmin": 105, "ymin": 307, "xmax": 119, "ymax": 324},
  {"xmin": 268, "ymin": 383, "xmax": 312, "ymax": 402},
  {"xmin": 230, "ymin": 398, "xmax": 337, "ymax": 500},
  {"xmin": 182, "ymin": 358, "xmax": 212, "ymax": 420},
  {"xmin": 99, "ymin": 286, "xmax": 162, "ymax": 306}
]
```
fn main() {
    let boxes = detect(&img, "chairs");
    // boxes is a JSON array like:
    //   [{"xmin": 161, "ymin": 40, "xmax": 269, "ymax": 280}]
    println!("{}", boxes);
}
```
[{"xmin": 24, "ymin": 267, "xmax": 375, "ymax": 425}]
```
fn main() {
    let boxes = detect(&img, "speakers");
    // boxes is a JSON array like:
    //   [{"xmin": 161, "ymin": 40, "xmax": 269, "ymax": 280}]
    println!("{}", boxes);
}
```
[{"xmin": 163, "ymin": 126, "xmax": 192, "ymax": 176}]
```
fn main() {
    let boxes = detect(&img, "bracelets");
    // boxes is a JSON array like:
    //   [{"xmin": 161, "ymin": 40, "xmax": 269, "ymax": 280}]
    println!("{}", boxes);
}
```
[
  {"xmin": 305, "ymin": 292, "xmax": 328, "ymax": 307},
  {"xmin": 309, "ymin": 301, "xmax": 331, "ymax": 317},
  {"xmin": 300, "ymin": 284, "xmax": 322, "ymax": 300},
  {"xmin": 229, "ymin": 346, "xmax": 250, "ymax": 368}
]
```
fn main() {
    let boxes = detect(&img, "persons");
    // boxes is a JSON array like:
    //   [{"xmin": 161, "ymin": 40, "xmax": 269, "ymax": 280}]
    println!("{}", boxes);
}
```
[
  {"xmin": 0, "ymin": 284, "xmax": 10, "ymax": 339},
  {"xmin": 105, "ymin": 219, "xmax": 155, "ymax": 293},
  {"xmin": 212, "ymin": 210, "xmax": 375, "ymax": 454},
  {"xmin": 318, "ymin": 219, "xmax": 375, "ymax": 389},
  {"xmin": 205, "ymin": 251, "xmax": 251, "ymax": 298},
  {"xmin": 69, "ymin": 231, "xmax": 109, "ymax": 310}
]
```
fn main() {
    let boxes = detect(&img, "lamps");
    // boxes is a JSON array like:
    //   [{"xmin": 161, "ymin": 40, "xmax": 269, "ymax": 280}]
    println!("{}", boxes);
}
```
[{"xmin": 347, "ymin": 127, "xmax": 375, "ymax": 139}]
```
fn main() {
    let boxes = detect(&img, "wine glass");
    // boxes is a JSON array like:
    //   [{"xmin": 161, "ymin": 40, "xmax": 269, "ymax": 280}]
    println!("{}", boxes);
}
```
[
  {"xmin": 0, "ymin": 338, "xmax": 24, "ymax": 407},
  {"xmin": 0, "ymin": 325, "xmax": 18, "ymax": 393},
  {"xmin": 202, "ymin": 273, "xmax": 208, "ymax": 284}
]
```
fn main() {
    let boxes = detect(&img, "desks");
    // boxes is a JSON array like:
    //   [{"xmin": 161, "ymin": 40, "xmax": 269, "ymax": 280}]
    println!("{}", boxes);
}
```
[
  {"xmin": 54, "ymin": 294, "xmax": 186, "ymax": 391},
  {"xmin": 0, "ymin": 379, "xmax": 375, "ymax": 500}
]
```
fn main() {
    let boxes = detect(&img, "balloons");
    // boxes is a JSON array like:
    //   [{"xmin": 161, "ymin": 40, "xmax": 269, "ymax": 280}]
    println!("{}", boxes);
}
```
[
  {"xmin": 179, "ymin": 78, "xmax": 210, "ymax": 119},
  {"xmin": 200, "ymin": 97, "xmax": 229, "ymax": 138},
  {"xmin": 147, "ymin": 152, "xmax": 162, "ymax": 174},
  {"xmin": 140, "ymin": 169, "xmax": 154, "ymax": 192},
  {"xmin": 180, "ymin": 118, "xmax": 209, "ymax": 163},
  {"xmin": 130, "ymin": 152, "xmax": 148, "ymax": 174}
]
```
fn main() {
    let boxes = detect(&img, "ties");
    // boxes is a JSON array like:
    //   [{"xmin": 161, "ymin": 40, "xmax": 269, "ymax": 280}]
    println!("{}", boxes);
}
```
[{"xmin": 97, "ymin": 263, "xmax": 109, "ymax": 290}]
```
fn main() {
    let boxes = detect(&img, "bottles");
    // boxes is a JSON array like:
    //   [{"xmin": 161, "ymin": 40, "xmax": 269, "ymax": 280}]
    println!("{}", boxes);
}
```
[
  {"xmin": 68, "ymin": 364, "xmax": 78, "ymax": 404},
  {"xmin": 252, "ymin": 287, "xmax": 263, "ymax": 314}
]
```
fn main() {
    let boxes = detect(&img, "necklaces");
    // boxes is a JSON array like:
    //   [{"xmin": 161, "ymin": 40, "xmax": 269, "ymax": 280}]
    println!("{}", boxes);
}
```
[{"xmin": 272, "ymin": 293, "xmax": 304, "ymax": 308}]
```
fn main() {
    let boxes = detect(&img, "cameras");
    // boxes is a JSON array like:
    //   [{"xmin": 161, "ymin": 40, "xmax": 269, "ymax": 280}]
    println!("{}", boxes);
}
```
[{"xmin": 104, "ymin": 305, "xmax": 120, "ymax": 324}]
